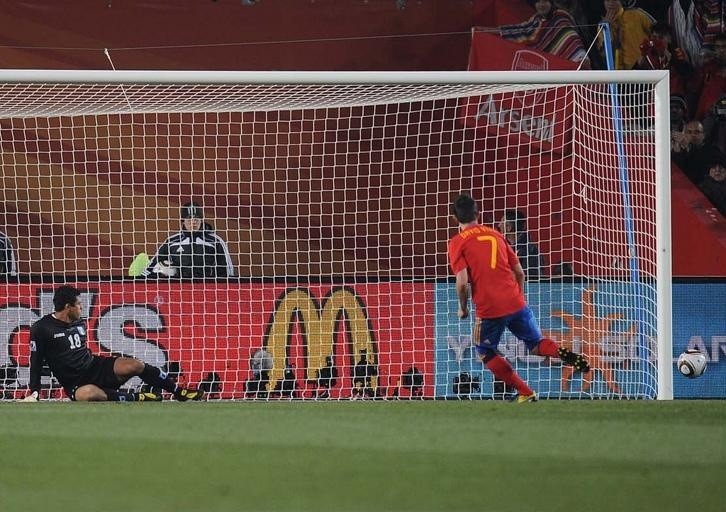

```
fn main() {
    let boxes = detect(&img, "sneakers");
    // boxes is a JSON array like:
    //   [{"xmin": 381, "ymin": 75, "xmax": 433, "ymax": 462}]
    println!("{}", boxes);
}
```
[
  {"xmin": 175, "ymin": 388, "xmax": 204, "ymax": 402},
  {"xmin": 557, "ymin": 347, "xmax": 590, "ymax": 373},
  {"xmin": 132, "ymin": 392, "xmax": 163, "ymax": 401},
  {"xmin": 518, "ymin": 389, "xmax": 538, "ymax": 402}
]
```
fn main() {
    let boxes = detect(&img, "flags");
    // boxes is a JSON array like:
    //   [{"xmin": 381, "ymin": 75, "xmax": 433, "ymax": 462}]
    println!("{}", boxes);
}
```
[{"xmin": 459, "ymin": 28, "xmax": 587, "ymax": 156}]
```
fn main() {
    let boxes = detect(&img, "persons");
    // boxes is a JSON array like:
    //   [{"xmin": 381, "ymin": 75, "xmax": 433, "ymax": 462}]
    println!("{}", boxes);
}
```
[
  {"xmin": 14, "ymin": 285, "xmax": 206, "ymax": 402},
  {"xmin": 448, "ymin": 194, "xmax": 591, "ymax": 403},
  {"xmin": 477, "ymin": 1, "xmax": 590, "ymax": 68},
  {"xmin": 497, "ymin": 212, "xmax": 545, "ymax": 274},
  {"xmin": 141, "ymin": 202, "xmax": 234, "ymax": 277},
  {"xmin": 594, "ymin": 1, "xmax": 726, "ymax": 215},
  {"xmin": 0, "ymin": 230, "xmax": 17, "ymax": 276}
]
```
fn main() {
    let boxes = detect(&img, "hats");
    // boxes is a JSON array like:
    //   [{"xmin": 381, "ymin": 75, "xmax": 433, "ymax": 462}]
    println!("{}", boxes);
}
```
[{"xmin": 179, "ymin": 202, "xmax": 203, "ymax": 219}]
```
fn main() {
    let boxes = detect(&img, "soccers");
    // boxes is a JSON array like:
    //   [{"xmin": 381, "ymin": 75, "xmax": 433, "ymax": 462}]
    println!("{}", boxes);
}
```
[
  {"xmin": 251, "ymin": 351, "xmax": 273, "ymax": 374},
  {"xmin": 676, "ymin": 349, "xmax": 706, "ymax": 379}
]
